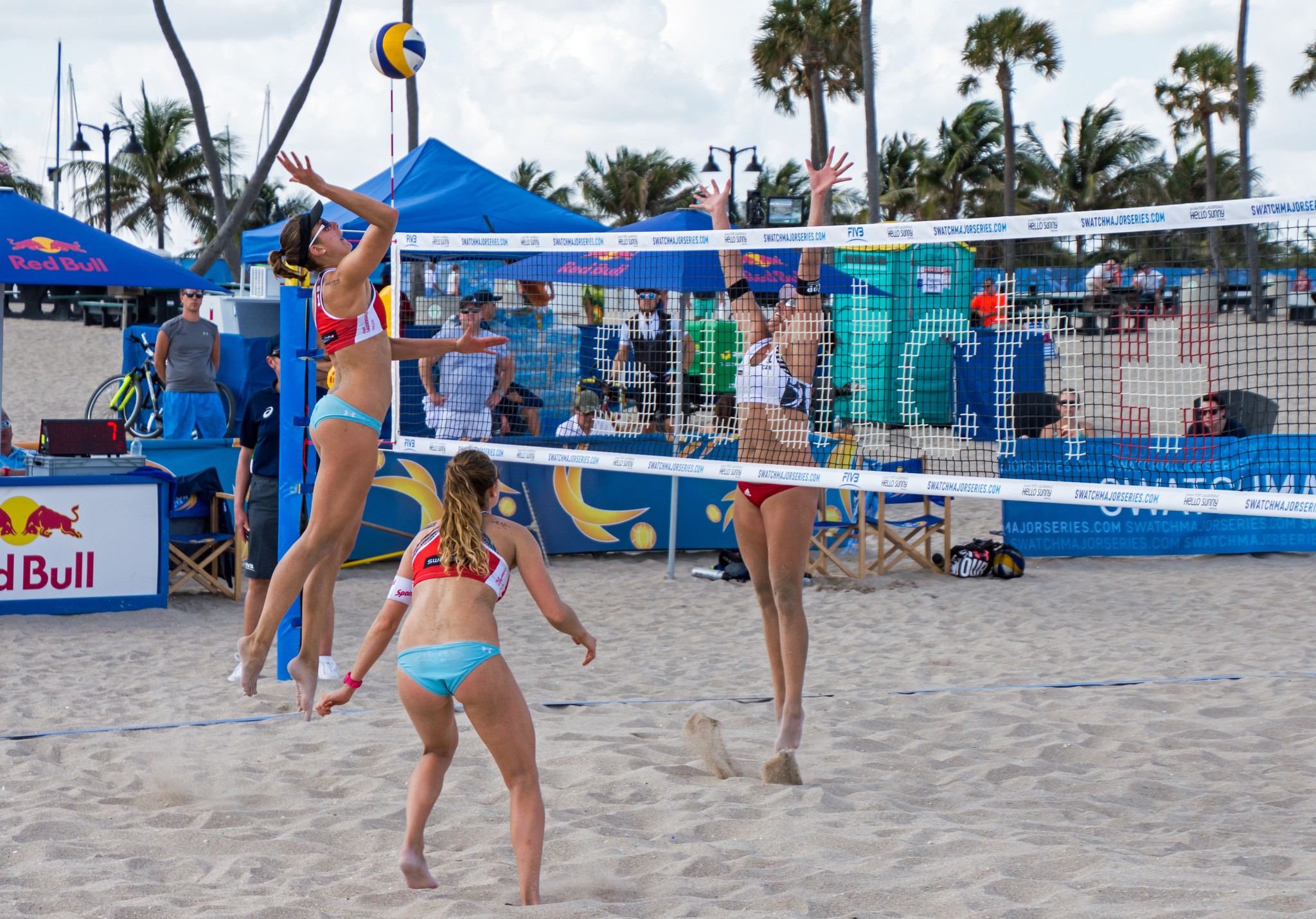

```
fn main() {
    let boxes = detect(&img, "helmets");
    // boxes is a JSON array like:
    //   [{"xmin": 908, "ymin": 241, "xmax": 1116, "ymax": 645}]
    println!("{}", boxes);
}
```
[{"xmin": 635, "ymin": 287, "xmax": 660, "ymax": 294}]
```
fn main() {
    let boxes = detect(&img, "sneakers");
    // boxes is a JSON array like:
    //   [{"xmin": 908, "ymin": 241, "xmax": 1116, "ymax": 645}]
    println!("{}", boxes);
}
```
[
  {"xmin": 227, "ymin": 653, "xmax": 242, "ymax": 682},
  {"xmin": 318, "ymin": 656, "xmax": 340, "ymax": 680}
]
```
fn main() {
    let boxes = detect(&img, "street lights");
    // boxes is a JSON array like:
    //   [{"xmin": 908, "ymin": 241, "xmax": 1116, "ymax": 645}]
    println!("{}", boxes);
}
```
[
  {"xmin": 67, "ymin": 121, "xmax": 146, "ymax": 238},
  {"xmin": 700, "ymin": 144, "xmax": 765, "ymax": 223}
]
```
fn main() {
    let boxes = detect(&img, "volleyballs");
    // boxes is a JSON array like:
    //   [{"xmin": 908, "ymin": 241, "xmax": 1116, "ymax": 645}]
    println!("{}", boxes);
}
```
[
  {"xmin": 369, "ymin": 22, "xmax": 426, "ymax": 79},
  {"xmin": 993, "ymin": 546, "xmax": 1024, "ymax": 576}
]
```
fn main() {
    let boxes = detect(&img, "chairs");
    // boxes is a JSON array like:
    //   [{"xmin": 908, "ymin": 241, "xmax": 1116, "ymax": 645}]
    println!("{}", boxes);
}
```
[
  {"xmin": 1193, "ymin": 388, "xmax": 1281, "ymax": 438},
  {"xmin": 867, "ymin": 454, "xmax": 954, "ymax": 577},
  {"xmin": 166, "ymin": 466, "xmax": 244, "ymax": 604},
  {"xmin": 804, "ymin": 487, "xmax": 866, "ymax": 580},
  {"xmin": 1001, "ymin": 391, "xmax": 1061, "ymax": 439}
]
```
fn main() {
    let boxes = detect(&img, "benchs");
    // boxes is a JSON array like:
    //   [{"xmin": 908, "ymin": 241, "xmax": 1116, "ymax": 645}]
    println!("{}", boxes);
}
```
[
  {"xmin": 1009, "ymin": 281, "xmax": 1316, "ymax": 336},
  {"xmin": 78, "ymin": 301, "xmax": 135, "ymax": 330}
]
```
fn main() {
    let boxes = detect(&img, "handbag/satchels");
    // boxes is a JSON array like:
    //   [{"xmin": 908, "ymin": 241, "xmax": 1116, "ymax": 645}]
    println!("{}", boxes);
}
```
[{"xmin": 950, "ymin": 538, "xmax": 1002, "ymax": 579}]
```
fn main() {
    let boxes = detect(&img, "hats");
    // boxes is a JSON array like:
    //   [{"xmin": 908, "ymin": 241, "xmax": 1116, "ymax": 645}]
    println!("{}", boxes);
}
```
[
  {"xmin": 472, "ymin": 289, "xmax": 503, "ymax": 306},
  {"xmin": 458, "ymin": 295, "xmax": 481, "ymax": 310},
  {"xmin": 297, "ymin": 199, "xmax": 324, "ymax": 267},
  {"xmin": 1140, "ymin": 263, "xmax": 1148, "ymax": 269},
  {"xmin": 266, "ymin": 332, "xmax": 280, "ymax": 356},
  {"xmin": 575, "ymin": 389, "xmax": 604, "ymax": 414},
  {"xmin": 779, "ymin": 283, "xmax": 798, "ymax": 300}
]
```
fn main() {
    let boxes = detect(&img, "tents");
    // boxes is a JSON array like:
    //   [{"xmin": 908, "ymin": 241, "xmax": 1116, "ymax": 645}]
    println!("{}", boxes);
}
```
[{"xmin": 237, "ymin": 136, "xmax": 605, "ymax": 302}]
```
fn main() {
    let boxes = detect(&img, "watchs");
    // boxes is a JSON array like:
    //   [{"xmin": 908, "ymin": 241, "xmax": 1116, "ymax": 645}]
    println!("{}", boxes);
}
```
[
  {"xmin": 497, "ymin": 389, "xmax": 504, "ymax": 397},
  {"xmin": 3, "ymin": 466, "xmax": 10, "ymax": 476},
  {"xmin": 343, "ymin": 672, "xmax": 363, "ymax": 688}
]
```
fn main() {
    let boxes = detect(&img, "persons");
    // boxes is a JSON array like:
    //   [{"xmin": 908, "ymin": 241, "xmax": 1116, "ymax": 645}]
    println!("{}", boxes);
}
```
[
  {"xmin": 0, "ymin": 407, "xmax": 40, "ymax": 477},
  {"xmin": 151, "ymin": 288, "xmax": 228, "ymax": 442},
  {"xmin": 686, "ymin": 145, "xmax": 855, "ymax": 756},
  {"xmin": 228, "ymin": 337, "xmax": 343, "ymax": 684},
  {"xmin": 417, "ymin": 261, "xmax": 721, "ymax": 438},
  {"xmin": 1290, "ymin": 268, "xmax": 1314, "ymax": 292},
  {"xmin": 317, "ymin": 452, "xmax": 595, "ymax": 904},
  {"xmin": 239, "ymin": 149, "xmax": 510, "ymax": 721},
  {"xmin": 969, "ymin": 256, "xmax": 1250, "ymax": 437}
]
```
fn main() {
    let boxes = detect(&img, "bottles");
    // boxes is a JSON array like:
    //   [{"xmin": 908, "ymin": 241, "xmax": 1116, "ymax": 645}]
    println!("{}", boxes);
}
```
[
  {"xmin": 691, "ymin": 567, "xmax": 732, "ymax": 582},
  {"xmin": 834, "ymin": 415, "xmax": 842, "ymax": 431},
  {"xmin": 932, "ymin": 553, "xmax": 944, "ymax": 572},
  {"xmin": 130, "ymin": 437, "xmax": 142, "ymax": 456}
]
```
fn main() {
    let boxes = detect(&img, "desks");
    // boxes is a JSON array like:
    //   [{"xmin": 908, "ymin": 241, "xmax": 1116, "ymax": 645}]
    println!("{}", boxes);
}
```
[
  {"xmin": 1107, "ymin": 286, "xmax": 1144, "ymax": 302},
  {"xmin": 1218, "ymin": 282, "xmax": 1272, "ymax": 290},
  {"xmin": 1012, "ymin": 294, "xmax": 1087, "ymax": 304},
  {"xmin": 0, "ymin": 454, "xmax": 170, "ymax": 615}
]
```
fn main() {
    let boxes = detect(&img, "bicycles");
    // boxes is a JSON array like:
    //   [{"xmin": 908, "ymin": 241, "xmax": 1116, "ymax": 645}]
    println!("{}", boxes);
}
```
[{"xmin": 85, "ymin": 333, "xmax": 236, "ymax": 440}]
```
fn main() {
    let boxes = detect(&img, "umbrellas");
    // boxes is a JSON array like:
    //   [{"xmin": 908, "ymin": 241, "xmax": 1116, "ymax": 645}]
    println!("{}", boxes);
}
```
[
  {"xmin": 0, "ymin": 186, "xmax": 236, "ymax": 409},
  {"xmin": 472, "ymin": 204, "xmax": 898, "ymax": 299}
]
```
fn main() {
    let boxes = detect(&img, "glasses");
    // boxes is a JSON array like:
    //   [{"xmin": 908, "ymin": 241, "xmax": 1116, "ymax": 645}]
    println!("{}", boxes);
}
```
[
  {"xmin": 1141, "ymin": 266, "xmax": 1149, "ymax": 271},
  {"xmin": 982, "ymin": 284, "xmax": 994, "ymax": 288},
  {"xmin": 1057, "ymin": 400, "xmax": 1080, "ymax": 407},
  {"xmin": 780, "ymin": 298, "xmax": 797, "ymax": 308},
  {"xmin": 269, "ymin": 352, "xmax": 280, "ymax": 358},
  {"xmin": 577, "ymin": 410, "xmax": 599, "ymax": 416},
  {"xmin": 638, "ymin": 293, "xmax": 657, "ymax": 299},
  {"xmin": 1, "ymin": 420, "xmax": 10, "ymax": 428},
  {"xmin": 460, "ymin": 307, "xmax": 479, "ymax": 314},
  {"xmin": 308, "ymin": 218, "xmax": 332, "ymax": 251},
  {"xmin": 1205, "ymin": 271, "xmax": 1209, "ymax": 272},
  {"xmin": 1198, "ymin": 408, "xmax": 1220, "ymax": 416},
  {"xmin": 183, "ymin": 291, "xmax": 203, "ymax": 298}
]
